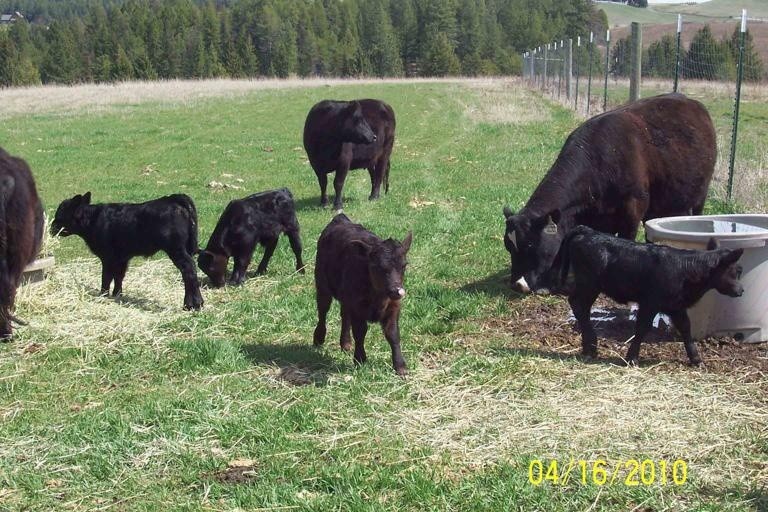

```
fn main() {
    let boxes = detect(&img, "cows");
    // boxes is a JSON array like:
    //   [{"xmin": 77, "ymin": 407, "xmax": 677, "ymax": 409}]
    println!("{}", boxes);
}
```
[
  {"xmin": 193, "ymin": 187, "xmax": 305, "ymax": 289},
  {"xmin": 303, "ymin": 99, "xmax": 396, "ymax": 217},
  {"xmin": 502, "ymin": 92, "xmax": 718, "ymax": 294},
  {"xmin": 49, "ymin": 191, "xmax": 204, "ymax": 311},
  {"xmin": 541, "ymin": 225, "xmax": 744, "ymax": 368},
  {"xmin": 0, "ymin": 147, "xmax": 44, "ymax": 338},
  {"xmin": 313, "ymin": 213, "xmax": 412, "ymax": 379}
]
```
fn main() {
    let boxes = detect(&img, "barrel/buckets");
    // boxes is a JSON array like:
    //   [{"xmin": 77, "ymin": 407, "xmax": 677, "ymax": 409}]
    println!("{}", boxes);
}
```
[{"xmin": 646, "ymin": 214, "xmax": 767, "ymax": 343}]
